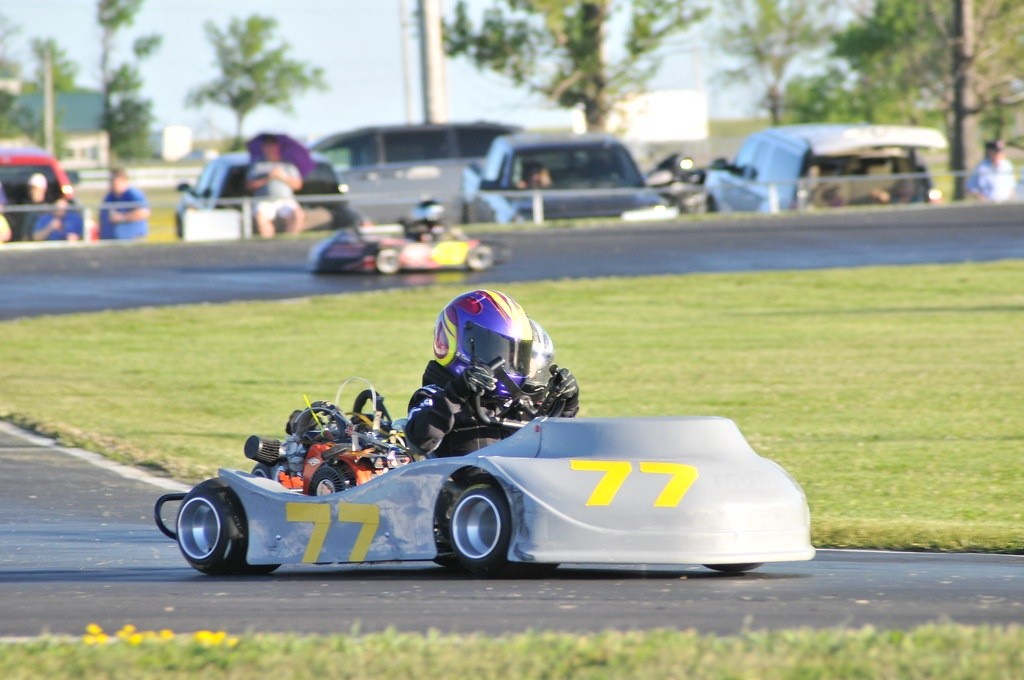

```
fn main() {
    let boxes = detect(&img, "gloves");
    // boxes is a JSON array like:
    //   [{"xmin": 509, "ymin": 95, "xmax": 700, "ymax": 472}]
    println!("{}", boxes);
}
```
[
  {"xmin": 453, "ymin": 364, "xmax": 499, "ymax": 398},
  {"xmin": 545, "ymin": 364, "xmax": 580, "ymax": 418}
]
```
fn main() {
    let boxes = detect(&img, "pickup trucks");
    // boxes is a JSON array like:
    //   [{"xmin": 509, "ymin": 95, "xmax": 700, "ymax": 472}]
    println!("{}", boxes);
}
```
[{"xmin": 174, "ymin": 151, "xmax": 369, "ymax": 233}]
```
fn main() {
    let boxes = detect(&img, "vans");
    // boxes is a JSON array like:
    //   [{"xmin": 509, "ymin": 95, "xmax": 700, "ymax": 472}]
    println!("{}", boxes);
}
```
[{"xmin": 315, "ymin": 119, "xmax": 526, "ymax": 227}]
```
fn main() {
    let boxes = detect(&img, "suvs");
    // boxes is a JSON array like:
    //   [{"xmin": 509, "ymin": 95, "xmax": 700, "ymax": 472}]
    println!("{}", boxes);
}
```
[
  {"xmin": 1, "ymin": 143, "xmax": 99, "ymax": 242},
  {"xmin": 705, "ymin": 123, "xmax": 950, "ymax": 213}
]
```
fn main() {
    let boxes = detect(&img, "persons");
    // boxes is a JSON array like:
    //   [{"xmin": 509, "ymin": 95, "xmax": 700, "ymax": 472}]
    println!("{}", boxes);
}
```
[
  {"xmin": 402, "ymin": 199, "xmax": 444, "ymax": 243},
  {"xmin": 33, "ymin": 200, "xmax": 83, "ymax": 242},
  {"xmin": 0, "ymin": 203, "xmax": 12, "ymax": 243},
  {"xmin": 967, "ymin": 139, "xmax": 1016, "ymax": 203},
  {"xmin": 517, "ymin": 163, "xmax": 551, "ymax": 187},
  {"xmin": 99, "ymin": 168, "xmax": 149, "ymax": 239},
  {"xmin": 16, "ymin": 175, "xmax": 51, "ymax": 242},
  {"xmin": 403, "ymin": 289, "xmax": 579, "ymax": 458},
  {"xmin": 245, "ymin": 141, "xmax": 304, "ymax": 237}
]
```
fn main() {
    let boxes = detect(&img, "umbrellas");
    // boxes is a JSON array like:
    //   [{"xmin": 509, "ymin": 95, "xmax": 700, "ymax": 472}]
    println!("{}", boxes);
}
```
[{"xmin": 248, "ymin": 135, "xmax": 312, "ymax": 177}]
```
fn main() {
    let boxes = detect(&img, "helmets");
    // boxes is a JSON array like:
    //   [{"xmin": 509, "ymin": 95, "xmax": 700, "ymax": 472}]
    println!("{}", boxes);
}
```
[
  {"xmin": 432, "ymin": 289, "xmax": 555, "ymax": 400},
  {"xmin": 411, "ymin": 199, "xmax": 444, "ymax": 221}
]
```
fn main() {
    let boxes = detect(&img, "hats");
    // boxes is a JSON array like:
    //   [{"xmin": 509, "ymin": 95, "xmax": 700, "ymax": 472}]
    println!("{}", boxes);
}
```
[
  {"xmin": 26, "ymin": 173, "xmax": 48, "ymax": 188},
  {"xmin": 985, "ymin": 141, "xmax": 1004, "ymax": 151}
]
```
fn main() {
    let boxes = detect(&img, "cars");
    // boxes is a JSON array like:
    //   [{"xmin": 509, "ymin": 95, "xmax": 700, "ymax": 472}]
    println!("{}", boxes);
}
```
[
  {"xmin": 463, "ymin": 138, "xmax": 675, "ymax": 218},
  {"xmin": 645, "ymin": 151, "xmax": 711, "ymax": 210}
]
